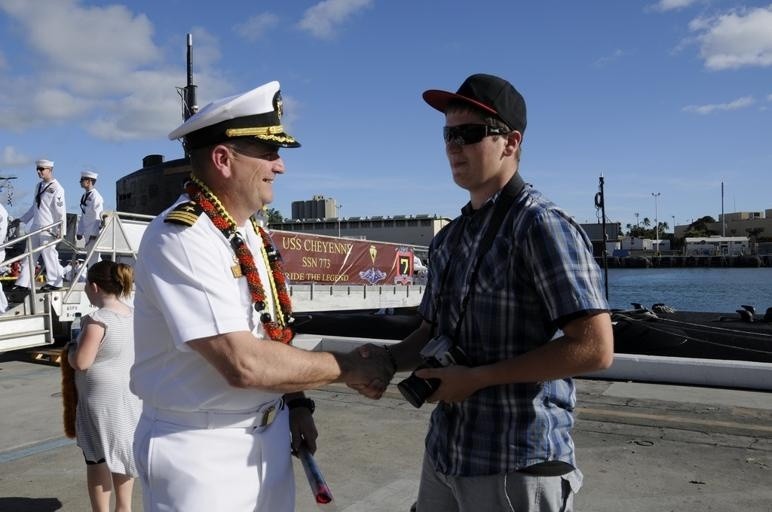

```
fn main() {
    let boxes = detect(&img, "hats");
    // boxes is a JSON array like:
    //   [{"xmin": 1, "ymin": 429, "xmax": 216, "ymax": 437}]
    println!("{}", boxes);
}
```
[
  {"xmin": 168, "ymin": 79, "xmax": 298, "ymax": 148},
  {"xmin": 81, "ymin": 171, "xmax": 98, "ymax": 182},
  {"xmin": 36, "ymin": 160, "xmax": 54, "ymax": 167},
  {"xmin": 422, "ymin": 73, "xmax": 527, "ymax": 133}
]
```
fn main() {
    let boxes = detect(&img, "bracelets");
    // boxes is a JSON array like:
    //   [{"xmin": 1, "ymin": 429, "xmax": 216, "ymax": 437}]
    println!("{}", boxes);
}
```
[
  {"xmin": 287, "ymin": 396, "xmax": 317, "ymax": 413},
  {"xmin": 383, "ymin": 344, "xmax": 399, "ymax": 372}
]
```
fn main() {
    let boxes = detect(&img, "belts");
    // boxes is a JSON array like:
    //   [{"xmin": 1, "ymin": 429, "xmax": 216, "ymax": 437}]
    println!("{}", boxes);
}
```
[{"xmin": 143, "ymin": 403, "xmax": 280, "ymax": 429}]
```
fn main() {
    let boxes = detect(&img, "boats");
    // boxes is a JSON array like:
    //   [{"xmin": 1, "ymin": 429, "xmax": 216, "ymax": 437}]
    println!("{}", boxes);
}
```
[{"xmin": 611, "ymin": 301, "xmax": 772, "ymax": 362}]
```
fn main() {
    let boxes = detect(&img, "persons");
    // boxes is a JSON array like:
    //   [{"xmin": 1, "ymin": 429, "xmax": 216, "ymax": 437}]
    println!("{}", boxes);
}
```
[
  {"xmin": 59, "ymin": 260, "xmax": 145, "ymax": 512},
  {"xmin": 77, "ymin": 170, "xmax": 105, "ymax": 272},
  {"xmin": 345, "ymin": 72, "xmax": 617, "ymax": 512},
  {"xmin": 0, "ymin": 257, "xmax": 90, "ymax": 283},
  {"xmin": 8, "ymin": 158, "xmax": 67, "ymax": 303},
  {"xmin": 127, "ymin": 79, "xmax": 398, "ymax": 511},
  {"xmin": 0, "ymin": 203, "xmax": 9, "ymax": 315}
]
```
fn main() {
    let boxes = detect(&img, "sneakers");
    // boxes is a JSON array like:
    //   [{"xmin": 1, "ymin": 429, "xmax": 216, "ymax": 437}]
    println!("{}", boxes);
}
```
[
  {"xmin": 41, "ymin": 284, "xmax": 59, "ymax": 291},
  {"xmin": 7, "ymin": 287, "xmax": 28, "ymax": 300}
]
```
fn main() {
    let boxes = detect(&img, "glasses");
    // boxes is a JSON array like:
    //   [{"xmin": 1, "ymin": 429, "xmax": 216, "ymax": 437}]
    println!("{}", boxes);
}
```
[
  {"xmin": 36, "ymin": 167, "xmax": 49, "ymax": 171},
  {"xmin": 443, "ymin": 124, "xmax": 506, "ymax": 145}
]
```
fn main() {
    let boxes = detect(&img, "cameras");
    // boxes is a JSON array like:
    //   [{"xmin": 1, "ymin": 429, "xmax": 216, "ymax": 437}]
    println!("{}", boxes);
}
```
[{"xmin": 397, "ymin": 334, "xmax": 469, "ymax": 408}]
